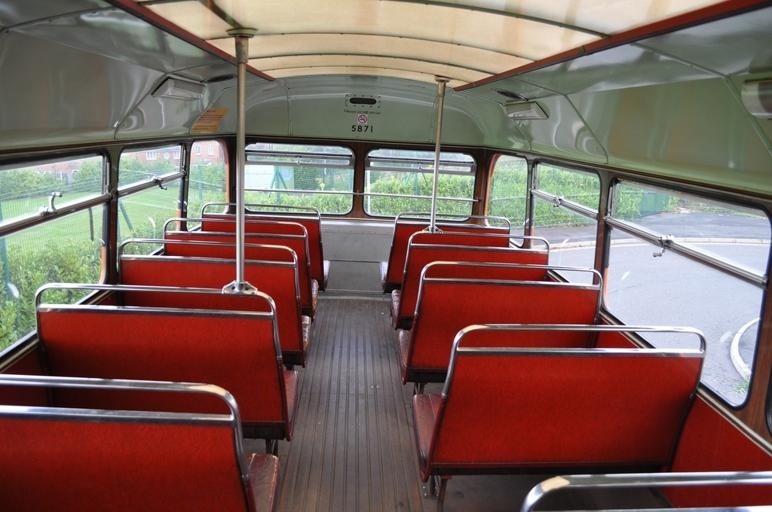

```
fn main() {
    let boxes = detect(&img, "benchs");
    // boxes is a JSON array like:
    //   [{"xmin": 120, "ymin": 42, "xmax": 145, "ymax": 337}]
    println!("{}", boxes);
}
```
[
  {"xmin": 162, "ymin": 218, "xmax": 319, "ymax": 319},
  {"xmin": 115, "ymin": 239, "xmax": 311, "ymax": 368},
  {"xmin": 2, "ymin": 374, "xmax": 278, "ymax": 511},
  {"xmin": 412, "ymin": 322, "xmax": 708, "ymax": 509},
  {"xmin": 36, "ymin": 283, "xmax": 300, "ymax": 454},
  {"xmin": 521, "ymin": 471, "xmax": 707, "ymax": 510},
  {"xmin": 390, "ymin": 232, "xmax": 549, "ymax": 330},
  {"xmin": 202, "ymin": 202, "xmax": 331, "ymax": 291},
  {"xmin": 379, "ymin": 213, "xmax": 511, "ymax": 296},
  {"xmin": 395, "ymin": 260, "xmax": 604, "ymax": 395}
]
